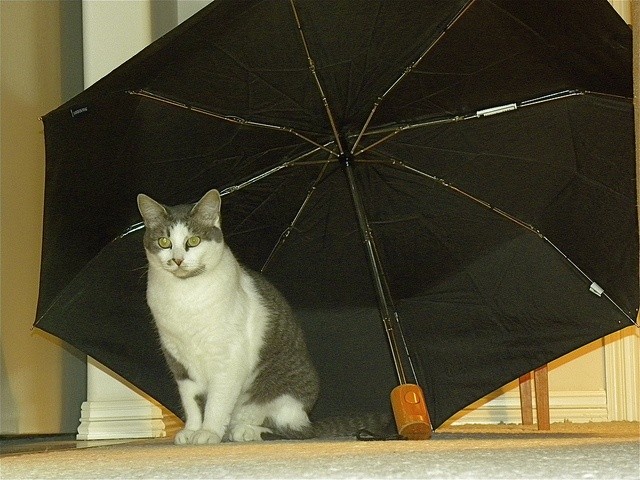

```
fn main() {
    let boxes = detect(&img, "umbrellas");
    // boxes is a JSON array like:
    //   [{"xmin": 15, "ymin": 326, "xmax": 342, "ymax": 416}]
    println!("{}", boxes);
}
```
[{"xmin": 33, "ymin": 0, "xmax": 640, "ymax": 441}]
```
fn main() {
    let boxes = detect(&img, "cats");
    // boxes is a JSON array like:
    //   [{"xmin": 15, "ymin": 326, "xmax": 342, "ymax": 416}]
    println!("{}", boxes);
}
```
[{"xmin": 129, "ymin": 188, "xmax": 393, "ymax": 445}]
